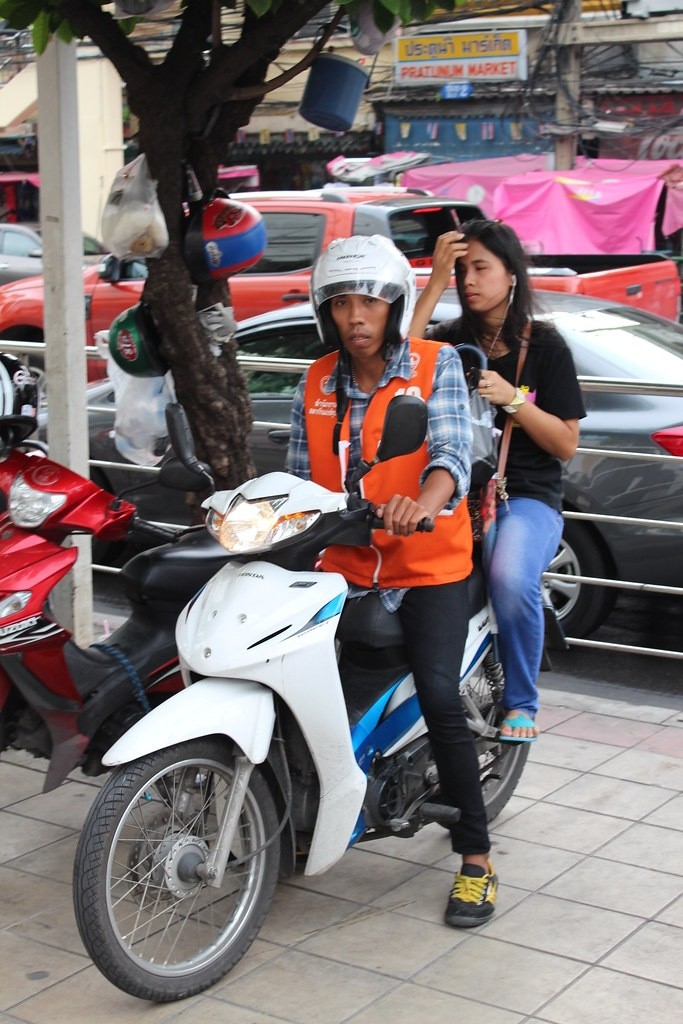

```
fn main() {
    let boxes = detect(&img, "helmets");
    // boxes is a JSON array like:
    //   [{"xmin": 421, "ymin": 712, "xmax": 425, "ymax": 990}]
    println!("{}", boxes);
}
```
[
  {"xmin": 308, "ymin": 234, "xmax": 416, "ymax": 344},
  {"xmin": 184, "ymin": 196, "xmax": 268, "ymax": 282},
  {"xmin": 108, "ymin": 301, "xmax": 172, "ymax": 378}
]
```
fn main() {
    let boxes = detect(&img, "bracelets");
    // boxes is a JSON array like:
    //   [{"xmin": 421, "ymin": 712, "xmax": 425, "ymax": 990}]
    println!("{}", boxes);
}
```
[{"xmin": 502, "ymin": 385, "xmax": 530, "ymax": 413}]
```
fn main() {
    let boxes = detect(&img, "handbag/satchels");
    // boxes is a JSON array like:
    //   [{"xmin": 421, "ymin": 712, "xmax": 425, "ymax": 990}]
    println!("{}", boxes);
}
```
[
  {"xmin": 100, "ymin": 151, "xmax": 169, "ymax": 261},
  {"xmin": 94, "ymin": 327, "xmax": 189, "ymax": 465},
  {"xmin": 454, "ymin": 343, "xmax": 493, "ymax": 464},
  {"xmin": 468, "ymin": 473, "xmax": 510, "ymax": 571}
]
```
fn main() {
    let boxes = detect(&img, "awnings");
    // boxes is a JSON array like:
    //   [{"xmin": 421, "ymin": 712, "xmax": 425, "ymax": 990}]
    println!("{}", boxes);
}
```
[
  {"xmin": 2, "ymin": 168, "xmax": 42, "ymax": 188},
  {"xmin": 401, "ymin": 152, "xmax": 682, "ymax": 262}
]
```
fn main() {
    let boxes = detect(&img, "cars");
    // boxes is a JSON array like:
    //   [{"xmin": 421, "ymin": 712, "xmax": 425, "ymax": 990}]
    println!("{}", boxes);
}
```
[
  {"xmin": 20, "ymin": 284, "xmax": 683, "ymax": 652},
  {"xmin": 0, "ymin": 221, "xmax": 112, "ymax": 287}
]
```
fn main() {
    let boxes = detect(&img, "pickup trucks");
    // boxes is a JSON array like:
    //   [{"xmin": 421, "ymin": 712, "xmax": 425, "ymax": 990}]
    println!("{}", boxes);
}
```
[{"xmin": 1, "ymin": 185, "xmax": 683, "ymax": 393}]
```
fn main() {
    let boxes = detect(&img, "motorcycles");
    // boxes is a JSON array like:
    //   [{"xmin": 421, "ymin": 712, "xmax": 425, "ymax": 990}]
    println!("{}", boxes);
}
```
[
  {"xmin": 71, "ymin": 401, "xmax": 567, "ymax": 1001},
  {"xmin": 1, "ymin": 413, "xmax": 252, "ymax": 793}
]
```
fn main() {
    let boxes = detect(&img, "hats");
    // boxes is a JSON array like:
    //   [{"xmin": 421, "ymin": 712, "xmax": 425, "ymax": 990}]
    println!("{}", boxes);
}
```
[{"xmin": 351, "ymin": 0, "xmax": 402, "ymax": 55}]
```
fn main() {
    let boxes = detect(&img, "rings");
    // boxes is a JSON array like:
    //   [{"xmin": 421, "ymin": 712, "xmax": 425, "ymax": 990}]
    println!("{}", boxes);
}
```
[{"xmin": 486, "ymin": 382, "xmax": 491, "ymax": 387}]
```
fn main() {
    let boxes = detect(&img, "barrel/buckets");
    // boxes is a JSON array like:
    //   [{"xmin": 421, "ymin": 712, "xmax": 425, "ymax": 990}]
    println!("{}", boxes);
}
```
[{"xmin": 298, "ymin": 21, "xmax": 379, "ymax": 131}]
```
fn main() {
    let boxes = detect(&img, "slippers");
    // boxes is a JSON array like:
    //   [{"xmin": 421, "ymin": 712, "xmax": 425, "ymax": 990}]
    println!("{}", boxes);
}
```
[{"xmin": 499, "ymin": 713, "xmax": 539, "ymax": 741}]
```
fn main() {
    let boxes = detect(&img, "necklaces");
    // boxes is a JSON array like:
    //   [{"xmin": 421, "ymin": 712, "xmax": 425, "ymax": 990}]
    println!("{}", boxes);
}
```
[{"xmin": 351, "ymin": 360, "xmax": 359, "ymax": 388}]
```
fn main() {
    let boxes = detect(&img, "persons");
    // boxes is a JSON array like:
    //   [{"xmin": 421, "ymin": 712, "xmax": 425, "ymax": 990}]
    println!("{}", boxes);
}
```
[
  {"xmin": 284, "ymin": 233, "xmax": 498, "ymax": 928},
  {"xmin": 408, "ymin": 220, "xmax": 588, "ymax": 744}
]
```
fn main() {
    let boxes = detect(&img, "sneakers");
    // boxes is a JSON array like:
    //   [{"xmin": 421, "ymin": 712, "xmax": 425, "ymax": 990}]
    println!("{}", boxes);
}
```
[{"xmin": 444, "ymin": 860, "xmax": 498, "ymax": 927}]
